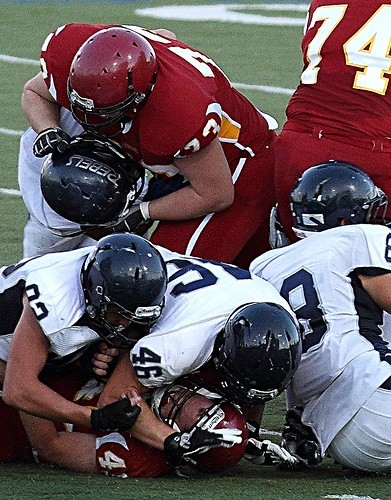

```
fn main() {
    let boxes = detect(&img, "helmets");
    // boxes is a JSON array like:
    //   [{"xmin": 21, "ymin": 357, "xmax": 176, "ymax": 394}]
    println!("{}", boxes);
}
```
[
  {"xmin": 67, "ymin": 27, "xmax": 158, "ymax": 137},
  {"xmin": 152, "ymin": 379, "xmax": 248, "ymax": 474},
  {"xmin": 40, "ymin": 128, "xmax": 144, "ymax": 225},
  {"xmin": 214, "ymin": 302, "xmax": 303, "ymax": 407},
  {"xmin": 289, "ymin": 161, "xmax": 389, "ymax": 239},
  {"xmin": 80, "ymin": 234, "xmax": 169, "ymax": 349}
]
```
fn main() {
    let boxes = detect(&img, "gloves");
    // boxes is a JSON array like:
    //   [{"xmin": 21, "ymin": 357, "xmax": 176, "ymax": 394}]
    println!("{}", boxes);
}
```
[
  {"xmin": 33, "ymin": 127, "xmax": 71, "ymax": 158},
  {"xmin": 164, "ymin": 427, "xmax": 243, "ymax": 478},
  {"xmin": 282, "ymin": 409, "xmax": 322, "ymax": 469},
  {"xmin": 248, "ymin": 419, "xmax": 298, "ymax": 468},
  {"xmin": 94, "ymin": 201, "xmax": 154, "ymax": 237}
]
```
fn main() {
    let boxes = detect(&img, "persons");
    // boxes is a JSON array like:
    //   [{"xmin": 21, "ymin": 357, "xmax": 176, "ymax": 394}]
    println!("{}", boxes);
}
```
[{"xmin": 1, "ymin": 1, "xmax": 390, "ymax": 478}]
